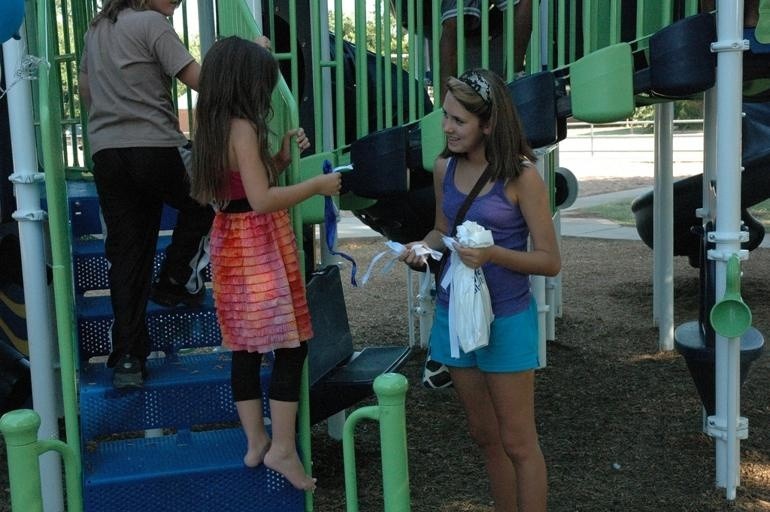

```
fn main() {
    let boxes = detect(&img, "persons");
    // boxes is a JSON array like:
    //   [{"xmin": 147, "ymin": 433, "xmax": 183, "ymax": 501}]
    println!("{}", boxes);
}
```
[
  {"xmin": 395, "ymin": 68, "xmax": 561, "ymax": 512},
  {"xmin": 186, "ymin": 33, "xmax": 342, "ymax": 492},
  {"xmin": 82, "ymin": 0, "xmax": 285, "ymax": 389}
]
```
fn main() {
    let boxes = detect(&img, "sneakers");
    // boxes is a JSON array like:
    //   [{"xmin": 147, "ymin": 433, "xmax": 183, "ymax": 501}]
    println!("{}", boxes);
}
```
[
  {"xmin": 110, "ymin": 354, "xmax": 151, "ymax": 392},
  {"xmin": 146, "ymin": 274, "xmax": 207, "ymax": 308}
]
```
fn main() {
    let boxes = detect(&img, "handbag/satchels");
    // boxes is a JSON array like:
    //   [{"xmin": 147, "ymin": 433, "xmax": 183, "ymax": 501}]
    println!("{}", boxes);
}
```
[{"xmin": 419, "ymin": 343, "xmax": 458, "ymax": 390}]
